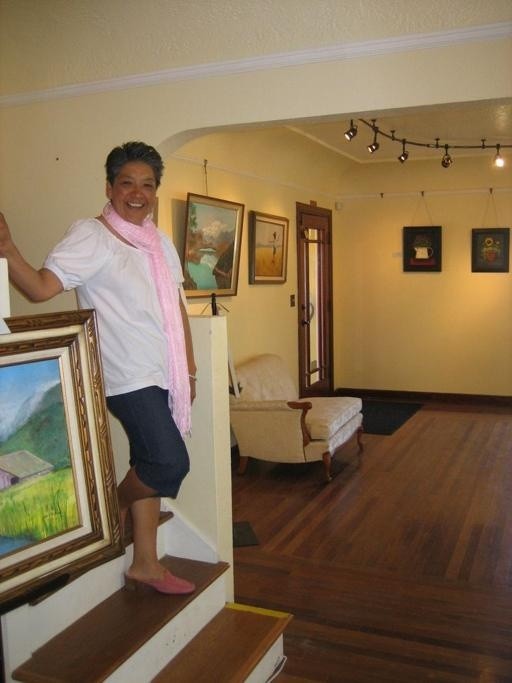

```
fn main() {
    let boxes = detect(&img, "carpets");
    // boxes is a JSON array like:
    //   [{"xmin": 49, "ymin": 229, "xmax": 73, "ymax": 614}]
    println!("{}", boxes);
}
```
[{"xmin": 358, "ymin": 396, "xmax": 426, "ymax": 435}]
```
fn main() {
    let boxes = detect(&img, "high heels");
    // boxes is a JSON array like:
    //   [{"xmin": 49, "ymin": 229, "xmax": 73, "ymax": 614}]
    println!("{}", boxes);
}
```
[{"xmin": 125, "ymin": 570, "xmax": 196, "ymax": 595}]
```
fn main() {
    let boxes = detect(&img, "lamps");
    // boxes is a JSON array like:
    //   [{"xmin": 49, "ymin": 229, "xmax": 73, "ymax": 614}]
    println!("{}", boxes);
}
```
[{"xmin": 344, "ymin": 117, "xmax": 512, "ymax": 169}]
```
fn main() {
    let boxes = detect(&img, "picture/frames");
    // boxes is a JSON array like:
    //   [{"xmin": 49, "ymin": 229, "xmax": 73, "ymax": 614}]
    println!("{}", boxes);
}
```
[
  {"xmin": 247, "ymin": 209, "xmax": 289, "ymax": 285},
  {"xmin": 184, "ymin": 192, "xmax": 245, "ymax": 297},
  {"xmin": 402, "ymin": 226, "xmax": 443, "ymax": 272},
  {"xmin": 470, "ymin": 227, "xmax": 510, "ymax": 272},
  {"xmin": 0, "ymin": 307, "xmax": 127, "ymax": 606}
]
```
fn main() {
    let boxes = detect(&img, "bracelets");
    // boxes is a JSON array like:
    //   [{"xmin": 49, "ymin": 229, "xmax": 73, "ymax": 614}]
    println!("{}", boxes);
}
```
[{"xmin": 189, "ymin": 375, "xmax": 197, "ymax": 381}]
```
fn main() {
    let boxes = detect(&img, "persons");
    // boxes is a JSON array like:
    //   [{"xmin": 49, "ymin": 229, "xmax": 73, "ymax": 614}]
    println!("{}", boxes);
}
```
[{"xmin": 0, "ymin": 141, "xmax": 198, "ymax": 598}]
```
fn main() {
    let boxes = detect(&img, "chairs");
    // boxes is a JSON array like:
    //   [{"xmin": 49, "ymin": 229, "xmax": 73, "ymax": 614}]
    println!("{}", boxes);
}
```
[{"xmin": 229, "ymin": 352, "xmax": 366, "ymax": 484}]
